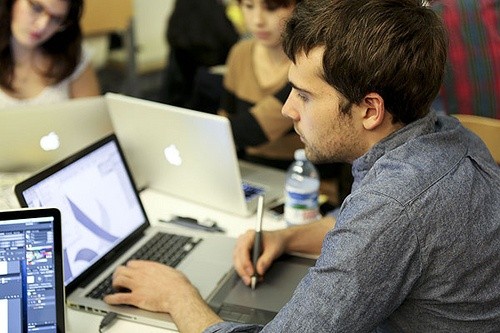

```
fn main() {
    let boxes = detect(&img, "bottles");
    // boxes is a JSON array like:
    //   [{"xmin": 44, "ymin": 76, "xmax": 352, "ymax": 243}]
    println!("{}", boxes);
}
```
[{"xmin": 284, "ymin": 148, "xmax": 321, "ymax": 226}]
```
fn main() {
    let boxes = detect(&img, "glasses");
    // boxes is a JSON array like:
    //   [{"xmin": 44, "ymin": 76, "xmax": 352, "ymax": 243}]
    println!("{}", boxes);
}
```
[{"xmin": 29, "ymin": 1, "xmax": 64, "ymax": 23}]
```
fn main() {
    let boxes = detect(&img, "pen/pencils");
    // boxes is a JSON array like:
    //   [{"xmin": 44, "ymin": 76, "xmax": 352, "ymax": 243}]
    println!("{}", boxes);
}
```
[{"xmin": 250, "ymin": 195, "xmax": 264, "ymax": 290}]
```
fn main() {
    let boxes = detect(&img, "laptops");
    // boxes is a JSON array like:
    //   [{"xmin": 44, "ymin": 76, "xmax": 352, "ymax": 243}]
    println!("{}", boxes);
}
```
[
  {"xmin": 106, "ymin": 92, "xmax": 291, "ymax": 217},
  {"xmin": 12, "ymin": 132, "xmax": 241, "ymax": 330},
  {"xmin": 0, "ymin": 94, "xmax": 114, "ymax": 175},
  {"xmin": 0, "ymin": 208, "xmax": 66, "ymax": 333}
]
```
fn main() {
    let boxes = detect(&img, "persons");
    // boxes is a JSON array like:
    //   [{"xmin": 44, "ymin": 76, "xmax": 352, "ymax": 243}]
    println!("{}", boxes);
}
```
[
  {"xmin": 104, "ymin": 0, "xmax": 500, "ymax": 333},
  {"xmin": 0, "ymin": 0, "xmax": 500, "ymax": 172}
]
